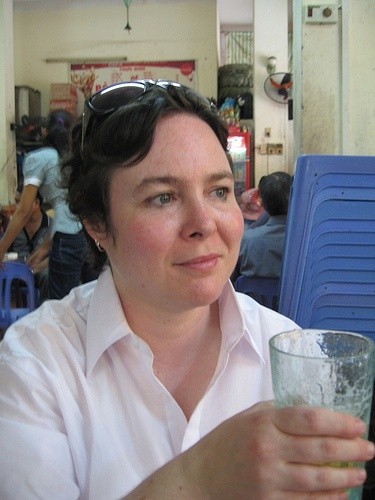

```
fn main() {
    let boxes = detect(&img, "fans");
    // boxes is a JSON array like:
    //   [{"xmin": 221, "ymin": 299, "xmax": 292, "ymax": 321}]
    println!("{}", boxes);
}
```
[{"xmin": 263, "ymin": 56, "xmax": 294, "ymax": 104}]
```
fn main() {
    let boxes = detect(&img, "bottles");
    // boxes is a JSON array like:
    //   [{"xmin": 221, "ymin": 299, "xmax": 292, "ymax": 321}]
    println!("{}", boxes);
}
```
[{"xmin": 217, "ymin": 104, "xmax": 240, "ymax": 118}]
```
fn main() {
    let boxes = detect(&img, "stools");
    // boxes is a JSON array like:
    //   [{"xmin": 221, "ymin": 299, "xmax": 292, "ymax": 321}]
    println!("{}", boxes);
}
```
[{"xmin": 276, "ymin": 154, "xmax": 375, "ymax": 345}]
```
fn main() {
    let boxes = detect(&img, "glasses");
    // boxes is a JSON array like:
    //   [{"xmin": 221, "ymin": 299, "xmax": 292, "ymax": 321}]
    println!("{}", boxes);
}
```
[{"xmin": 77, "ymin": 79, "xmax": 217, "ymax": 161}]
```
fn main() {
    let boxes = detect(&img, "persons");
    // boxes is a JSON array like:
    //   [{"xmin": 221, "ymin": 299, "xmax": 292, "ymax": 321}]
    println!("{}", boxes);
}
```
[
  {"xmin": 0, "ymin": 79, "xmax": 375, "ymax": 500},
  {"xmin": 0, "ymin": 107, "xmax": 294, "ymax": 299}
]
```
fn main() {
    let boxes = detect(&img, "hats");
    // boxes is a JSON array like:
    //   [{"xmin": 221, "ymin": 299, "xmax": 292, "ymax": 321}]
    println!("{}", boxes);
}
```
[{"xmin": 238, "ymin": 187, "xmax": 269, "ymax": 220}]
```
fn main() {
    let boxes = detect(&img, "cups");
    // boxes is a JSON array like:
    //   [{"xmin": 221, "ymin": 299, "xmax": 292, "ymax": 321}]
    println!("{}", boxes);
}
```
[{"xmin": 269, "ymin": 330, "xmax": 375, "ymax": 500}]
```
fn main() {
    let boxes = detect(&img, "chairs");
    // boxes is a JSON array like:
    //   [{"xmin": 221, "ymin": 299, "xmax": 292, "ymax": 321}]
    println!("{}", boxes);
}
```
[{"xmin": 0, "ymin": 262, "xmax": 34, "ymax": 329}]
